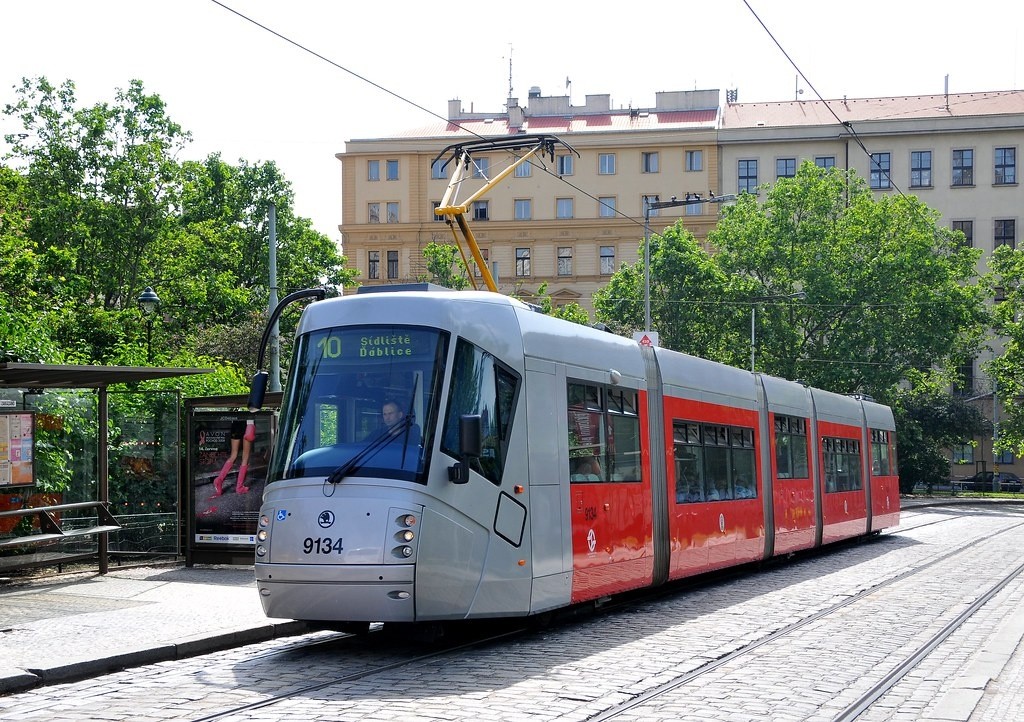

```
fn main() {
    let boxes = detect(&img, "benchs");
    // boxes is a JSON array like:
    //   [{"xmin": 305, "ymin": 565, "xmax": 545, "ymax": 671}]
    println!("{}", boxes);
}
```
[{"xmin": 0, "ymin": 502, "xmax": 122, "ymax": 546}]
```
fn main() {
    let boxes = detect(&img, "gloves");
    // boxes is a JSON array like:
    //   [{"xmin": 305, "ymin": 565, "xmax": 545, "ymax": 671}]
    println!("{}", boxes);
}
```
[{"xmin": 244, "ymin": 424, "xmax": 255, "ymax": 441}]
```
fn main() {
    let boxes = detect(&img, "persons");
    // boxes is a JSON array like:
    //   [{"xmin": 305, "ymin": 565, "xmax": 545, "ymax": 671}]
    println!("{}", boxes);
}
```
[
  {"xmin": 676, "ymin": 474, "xmax": 756, "ymax": 502},
  {"xmin": 212, "ymin": 418, "xmax": 255, "ymax": 495},
  {"xmin": 363, "ymin": 401, "xmax": 421, "ymax": 445}
]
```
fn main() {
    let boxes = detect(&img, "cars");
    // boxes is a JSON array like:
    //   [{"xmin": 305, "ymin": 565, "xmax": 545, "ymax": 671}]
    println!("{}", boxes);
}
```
[{"xmin": 957, "ymin": 471, "xmax": 1023, "ymax": 493}]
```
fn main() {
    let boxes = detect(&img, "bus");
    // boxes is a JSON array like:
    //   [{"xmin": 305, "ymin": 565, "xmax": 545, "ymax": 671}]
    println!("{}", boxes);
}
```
[{"xmin": 244, "ymin": 133, "xmax": 900, "ymax": 642}]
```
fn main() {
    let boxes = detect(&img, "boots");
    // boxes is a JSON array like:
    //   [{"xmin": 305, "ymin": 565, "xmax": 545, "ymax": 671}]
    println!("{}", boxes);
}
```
[
  {"xmin": 236, "ymin": 466, "xmax": 250, "ymax": 493},
  {"xmin": 215, "ymin": 462, "xmax": 233, "ymax": 494}
]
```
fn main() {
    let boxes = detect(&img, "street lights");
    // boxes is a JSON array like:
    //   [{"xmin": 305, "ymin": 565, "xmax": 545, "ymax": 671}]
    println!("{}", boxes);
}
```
[
  {"xmin": 137, "ymin": 286, "xmax": 161, "ymax": 365},
  {"xmin": 749, "ymin": 291, "xmax": 808, "ymax": 369},
  {"xmin": 644, "ymin": 194, "xmax": 739, "ymax": 332}
]
